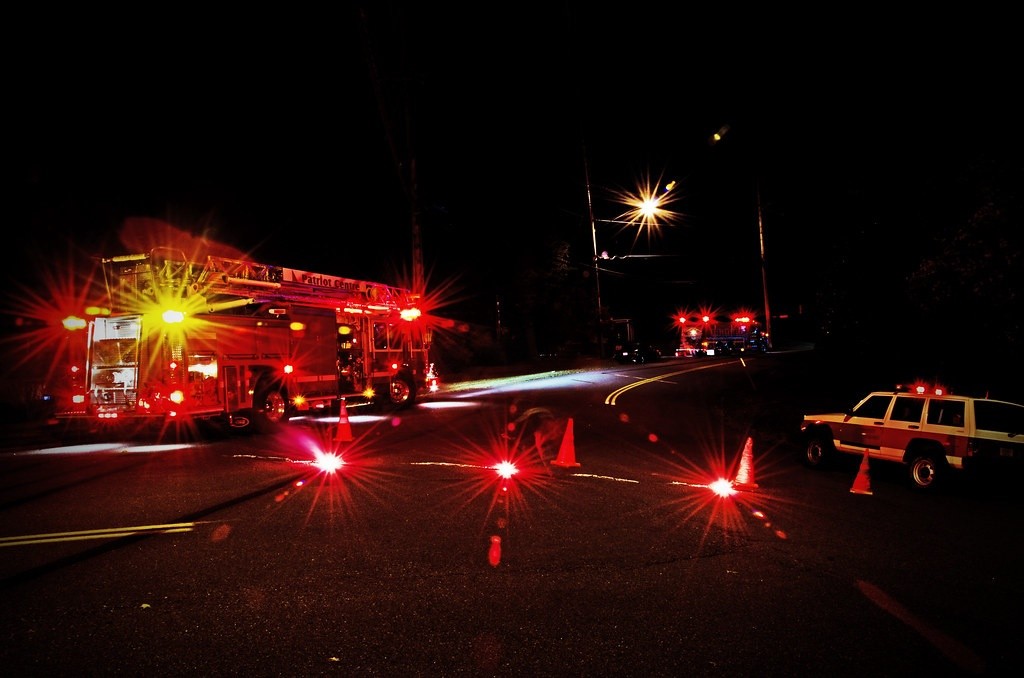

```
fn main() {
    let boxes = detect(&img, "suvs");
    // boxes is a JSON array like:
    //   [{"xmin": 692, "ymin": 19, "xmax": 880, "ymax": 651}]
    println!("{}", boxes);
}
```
[{"xmin": 799, "ymin": 383, "xmax": 1024, "ymax": 492}]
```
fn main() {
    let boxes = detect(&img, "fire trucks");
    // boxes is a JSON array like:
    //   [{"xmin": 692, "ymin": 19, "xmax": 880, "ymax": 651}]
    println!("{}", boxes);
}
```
[
  {"xmin": 674, "ymin": 314, "xmax": 768, "ymax": 358},
  {"xmin": 54, "ymin": 247, "xmax": 429, "ymax": 434}
]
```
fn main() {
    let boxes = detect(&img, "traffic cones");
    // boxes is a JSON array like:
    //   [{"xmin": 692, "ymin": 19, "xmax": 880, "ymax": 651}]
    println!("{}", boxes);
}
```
[
  {"xmin": 849, "ymin": 448, "xmax": 873, "ymax": 494},
  {"xmin": 333, "ymin": 399, "xmax": 356, "ymax": 442},
  {"xmin": 730, "ymin": 437, "xmax": 758, "ymax": 488},
  {"xmin": 550, "ymin": 418, "xmax": 580, "ymax": 466}
]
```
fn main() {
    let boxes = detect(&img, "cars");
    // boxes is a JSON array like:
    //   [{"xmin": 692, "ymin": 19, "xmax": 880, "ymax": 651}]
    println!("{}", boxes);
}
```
[{"xmin": 610, "ymin": 342, "xmax": 660, "ymax": 364}]
